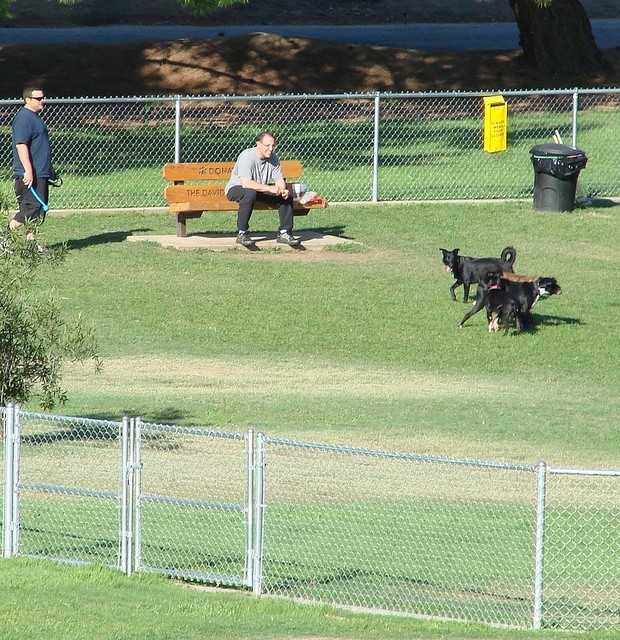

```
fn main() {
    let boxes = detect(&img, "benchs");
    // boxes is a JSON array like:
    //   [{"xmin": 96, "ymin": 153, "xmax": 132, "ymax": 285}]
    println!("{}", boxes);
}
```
[{"xmin": 161, "ymin": 160, "xmax": 328, "ymax": 237}]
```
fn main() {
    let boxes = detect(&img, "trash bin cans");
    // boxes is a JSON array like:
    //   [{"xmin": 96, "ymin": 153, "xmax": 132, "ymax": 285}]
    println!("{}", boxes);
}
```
[{"xmin": 529, "ymin": 144, "xmax": 588, "ymax": 212}]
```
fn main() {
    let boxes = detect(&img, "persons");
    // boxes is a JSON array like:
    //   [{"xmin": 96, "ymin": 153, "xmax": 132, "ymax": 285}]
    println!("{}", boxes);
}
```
[
  {"xmin": 223, "ymin": 132, "xmax": 300, "ymax": 247},
  {"xmin": 0, "ymin": 84, "xmax": 57, "ymax": 255}
]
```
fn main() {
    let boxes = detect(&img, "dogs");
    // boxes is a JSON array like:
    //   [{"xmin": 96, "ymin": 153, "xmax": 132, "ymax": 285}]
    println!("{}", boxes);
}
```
[
  {"xmin": 495, "ymin": 269, "xmax": 540, "ymax": 282},
  {"xmin": 439, "ymin": 247, "xmax": 518, "ymax": 304},
  {"xmin": 483, "ymin": 272, "xmax": 522, "ymax": 336},
  {"xmin": 458, "ymin": 276, "xmax": 562, "ymax": 330}
]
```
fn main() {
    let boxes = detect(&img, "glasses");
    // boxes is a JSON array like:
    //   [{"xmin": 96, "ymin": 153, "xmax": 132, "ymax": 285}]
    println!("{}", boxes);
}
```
[
  {"xmin": 259, "ymin": 140, "xmax": 275, "ymax": 148},
  {"xmin": 28, "ymin": 96, "xmax": 44, "ymax": 101}
]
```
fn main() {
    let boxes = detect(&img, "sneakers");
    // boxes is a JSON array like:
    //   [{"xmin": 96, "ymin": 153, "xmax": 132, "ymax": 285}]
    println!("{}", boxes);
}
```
[
  {"xmin": 277, "ymin": 231, "xmax": 301, "ymax": 246},
  {"xmin": 236, "ymin": 232, "xmax": 255, "ymax": 246}
]
